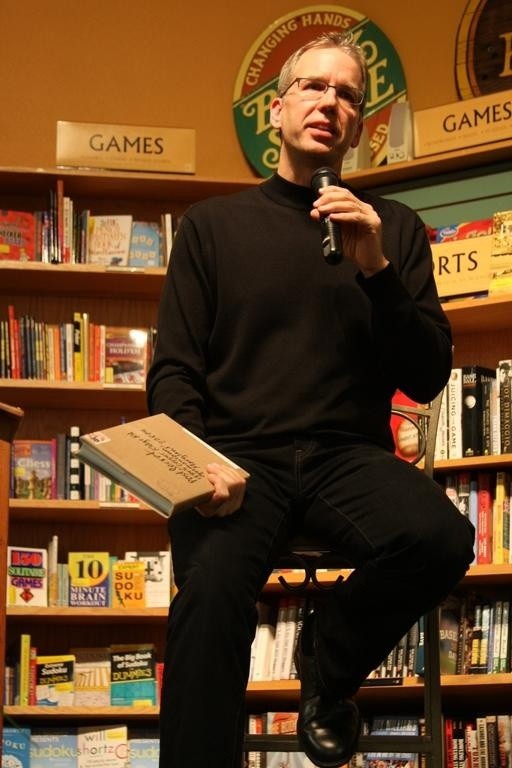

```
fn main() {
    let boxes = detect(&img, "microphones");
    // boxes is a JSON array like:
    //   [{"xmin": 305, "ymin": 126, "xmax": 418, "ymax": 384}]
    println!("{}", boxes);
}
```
[{"xmin": 309, "ymin": 166, "xmax": 345, "ymax": 265}]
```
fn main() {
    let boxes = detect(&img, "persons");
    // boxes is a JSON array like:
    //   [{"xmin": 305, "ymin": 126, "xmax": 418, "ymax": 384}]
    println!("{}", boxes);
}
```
[{"xmin": 146, "ymin": 36, "xmax": 478, "ymax": 766}]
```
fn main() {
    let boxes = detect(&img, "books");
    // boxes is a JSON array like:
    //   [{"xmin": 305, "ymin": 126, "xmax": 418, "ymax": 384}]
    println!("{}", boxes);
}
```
[{"xmin": 77, "ymin": 414, "xmax": 254, "ymax": 523}]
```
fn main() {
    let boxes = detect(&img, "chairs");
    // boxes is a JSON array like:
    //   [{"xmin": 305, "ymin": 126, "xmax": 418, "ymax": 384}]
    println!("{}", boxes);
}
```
[{"xmin": 225, "ymin": 365, "xmax": 452, "ymax": 766}]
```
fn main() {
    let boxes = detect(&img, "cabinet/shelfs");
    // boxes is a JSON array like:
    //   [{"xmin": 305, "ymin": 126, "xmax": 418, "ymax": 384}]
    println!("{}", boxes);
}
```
[
  {"xmin": 245, "ymin": 139, "xmax": 512, "ymax": 768},
  {"xmin": 0, "ymin": 162, "xmax": 265, "ymax": 768}
]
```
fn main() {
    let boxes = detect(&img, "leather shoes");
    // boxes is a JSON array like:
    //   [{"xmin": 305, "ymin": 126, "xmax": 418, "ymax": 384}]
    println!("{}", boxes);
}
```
[{"xmin": 290, "ymin": 610, "xmax": 362, "ymax": 768}]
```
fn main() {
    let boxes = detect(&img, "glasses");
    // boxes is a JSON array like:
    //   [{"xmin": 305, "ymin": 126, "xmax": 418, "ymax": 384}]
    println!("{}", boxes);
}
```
[{"xmin": 280, "ymin": 77, "xmax": 367, "ymax": 105}]
